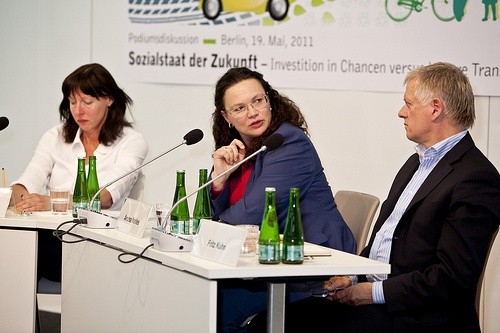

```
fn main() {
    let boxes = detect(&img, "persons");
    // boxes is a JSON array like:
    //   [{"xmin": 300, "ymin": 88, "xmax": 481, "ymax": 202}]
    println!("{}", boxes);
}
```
[
  {"xmin": 202, "ymin": 68, "xmax": 358, "ymax": 333},
  {"xmin": 238, "ymin": 61, "xmax": 500, "ymax": 333},
  {"xmin": 8, "ymin": 63, "xmax": 148, "ymax": 213}
]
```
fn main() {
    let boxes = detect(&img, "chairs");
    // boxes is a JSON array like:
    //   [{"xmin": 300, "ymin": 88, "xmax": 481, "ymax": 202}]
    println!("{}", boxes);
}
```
[{"xmin": 334, "ymin": 190, "xmax": 380, "ymax": 255}]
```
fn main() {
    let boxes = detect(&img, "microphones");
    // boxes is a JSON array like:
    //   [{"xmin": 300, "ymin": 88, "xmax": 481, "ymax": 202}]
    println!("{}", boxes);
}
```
[
  {"xmin": 77, "ymin": 129, "xmax": 204, "ymax": 230},
  {"xmin": 150, "ymin": 132, "xmax": 284, "ymax": 251},
  {"xmin": 0, "ymin": 116, "xmax": 10, "ymax": 131}
]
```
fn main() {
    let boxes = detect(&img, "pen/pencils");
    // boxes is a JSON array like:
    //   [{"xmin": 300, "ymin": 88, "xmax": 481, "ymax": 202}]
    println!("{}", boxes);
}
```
[{"xmin": 2, "ymin": 167, "xmax": 5, "ymax": 186}]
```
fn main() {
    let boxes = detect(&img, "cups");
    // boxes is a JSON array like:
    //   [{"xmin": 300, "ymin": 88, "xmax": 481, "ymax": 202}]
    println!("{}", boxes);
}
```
[
  {"xmin": 235, "ymin": 224, "xmax": 259, "ymax": 257},
  {"xmin": 49, "ymin": 187, "xmax": 70, "ymax": 215},
  {"xmin": 155, "ymin": 201, "xmax": 171, "ymax": 234}
]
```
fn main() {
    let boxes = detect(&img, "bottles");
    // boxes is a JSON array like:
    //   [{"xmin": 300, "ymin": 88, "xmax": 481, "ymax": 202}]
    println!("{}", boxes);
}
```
[
  {"xmin": 71, "ymin": 158, "xmax": 90, "ymax": 218},
  {"xmin": 192, "ymin": 168, "xmax": 213, "ymax": 235},
  {"xmin": 282, "ymin": 188, "xmax": 304, "ymax": 265},
  {"xmin": 170, "ymin": 169, "xmax": 190, "ymax": 235},
  {"xmin": 259, "ymin": 188, "xmax": 281, "ymax": 265},
  {"xmin": 86, "ymin": 156, "xmax": 101, "ymax": 212}
]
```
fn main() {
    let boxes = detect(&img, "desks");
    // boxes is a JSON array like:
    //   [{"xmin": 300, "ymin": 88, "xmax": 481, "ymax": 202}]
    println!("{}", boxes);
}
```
[{"xmin": 0, "ymin": 191, "xmax": 392, "ymax": 333}]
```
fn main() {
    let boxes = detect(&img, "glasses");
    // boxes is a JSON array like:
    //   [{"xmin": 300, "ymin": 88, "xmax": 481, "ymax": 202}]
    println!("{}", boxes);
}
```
[
  {"xmin": 311, "ymin": 287, "xmax": 346, "ymax": 298},
  {"xmin": 226, "ymin": 95, "xmax": 269, "ymax": 119}
]
